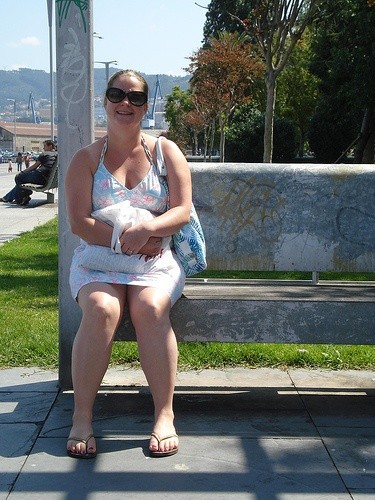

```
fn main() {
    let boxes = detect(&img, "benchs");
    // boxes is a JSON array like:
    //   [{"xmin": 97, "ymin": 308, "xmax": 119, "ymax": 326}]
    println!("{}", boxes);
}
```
[
  {"xmin": 20, "ymin": 155, "xmax": 59, "ymax": 204},
  {"xmin": 59, "ymin": 162, "xmax": 375, "ymax": 387}
]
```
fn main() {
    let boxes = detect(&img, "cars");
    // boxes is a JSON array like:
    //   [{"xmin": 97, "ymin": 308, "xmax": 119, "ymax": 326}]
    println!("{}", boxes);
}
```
[{"xmin": 0, "ymin": 149, "xmax": 42, "ymax": 162}]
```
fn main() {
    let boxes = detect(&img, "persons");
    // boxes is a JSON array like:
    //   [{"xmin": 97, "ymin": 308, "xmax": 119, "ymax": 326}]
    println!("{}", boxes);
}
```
[
  {"xmin": 67, "ymin": 70, "xmax": 191, "ymax": 459},
  {"xmin": 0, "ymin": 140, "xmax": 58, "ymax": 205}
]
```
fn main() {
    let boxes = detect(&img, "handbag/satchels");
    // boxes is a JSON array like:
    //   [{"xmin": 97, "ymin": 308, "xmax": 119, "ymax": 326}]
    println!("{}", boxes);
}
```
[{"xmin": 155, "ymin": 136, "xmax": 206, "ymax": 277}]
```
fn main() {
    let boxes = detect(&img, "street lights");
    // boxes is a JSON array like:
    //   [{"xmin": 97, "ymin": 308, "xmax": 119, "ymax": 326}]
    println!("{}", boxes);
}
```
[{"xmin": 7, "ymin": 98, "xmax": 17, "ymax": 155}]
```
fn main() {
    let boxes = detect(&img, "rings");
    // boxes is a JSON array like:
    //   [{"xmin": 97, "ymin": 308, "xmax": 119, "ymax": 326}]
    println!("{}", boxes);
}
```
[{"xmin": 128, "ymin": 250, "xmax": 133, "ymax": 253}]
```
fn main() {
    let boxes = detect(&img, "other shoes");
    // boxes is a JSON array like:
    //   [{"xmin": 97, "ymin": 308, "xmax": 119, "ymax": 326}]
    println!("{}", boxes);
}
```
[
  {"xmin": 0, "ymin": 198, "xmax": 8, "ymax": 203},
  {"xmin": 21, "ymin": 195, "xmax": 31, "ymax": 206}
]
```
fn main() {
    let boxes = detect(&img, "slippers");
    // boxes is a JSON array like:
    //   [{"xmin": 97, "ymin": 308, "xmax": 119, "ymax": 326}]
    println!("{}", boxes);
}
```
[
  {"xmin": 67, "ymin": 434, "xmax": 97, "ymax": 458},
  {"xmin": 149, "ymin": 432, "xmax": 179, "ymax": 457}
]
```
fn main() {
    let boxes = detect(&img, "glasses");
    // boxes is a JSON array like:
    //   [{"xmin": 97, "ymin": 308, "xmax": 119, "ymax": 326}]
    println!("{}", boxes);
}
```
[{"xmin": 106, "ymin": 88, "xmax": 147, "ymax": 107}]
